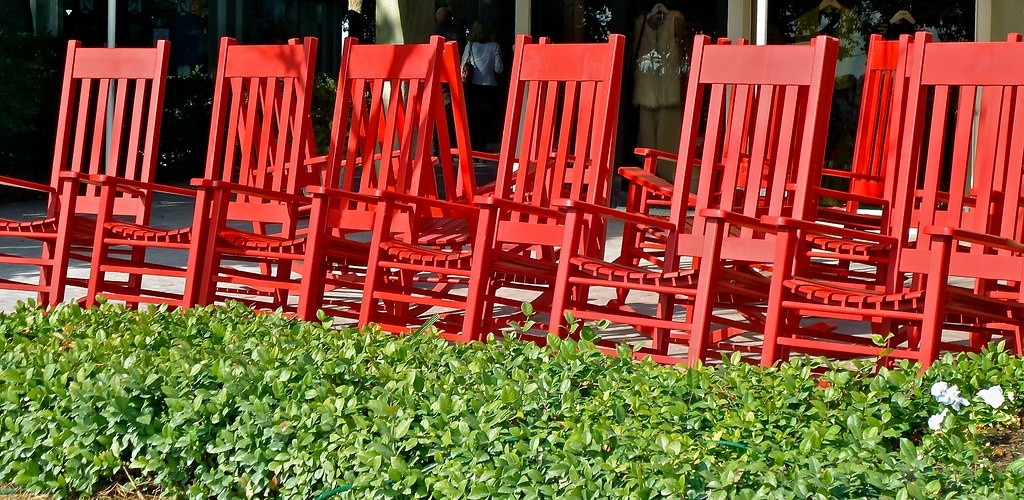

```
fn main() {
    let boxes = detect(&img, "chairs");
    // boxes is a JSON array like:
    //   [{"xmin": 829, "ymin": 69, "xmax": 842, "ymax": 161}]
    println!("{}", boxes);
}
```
[{"xmin": 1, "ymin": 32, "xmax": 1023, "ymax": 389}]
